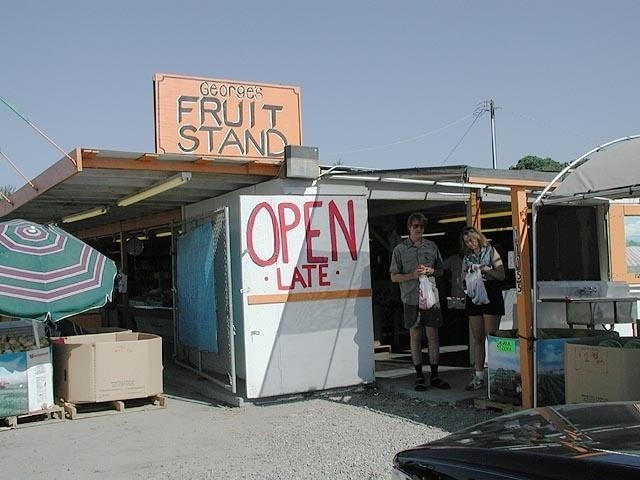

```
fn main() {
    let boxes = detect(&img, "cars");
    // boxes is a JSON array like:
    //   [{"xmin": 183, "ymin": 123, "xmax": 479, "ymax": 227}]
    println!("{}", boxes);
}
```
[{"xmin": 392, "ymin": 400, "xmax": 640, "ymax": 480}]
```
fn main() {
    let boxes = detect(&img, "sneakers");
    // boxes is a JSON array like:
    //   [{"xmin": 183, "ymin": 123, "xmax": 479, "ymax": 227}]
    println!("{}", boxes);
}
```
[{"xmin": 466, "ymin": 377, "xmax": 483, "ymax": 391}]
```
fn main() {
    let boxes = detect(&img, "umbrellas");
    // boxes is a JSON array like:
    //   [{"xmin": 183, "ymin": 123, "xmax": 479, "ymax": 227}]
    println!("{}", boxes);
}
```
[{"xmin": 0, "ymin": 219, "xmax": 117, "ymax": 322}]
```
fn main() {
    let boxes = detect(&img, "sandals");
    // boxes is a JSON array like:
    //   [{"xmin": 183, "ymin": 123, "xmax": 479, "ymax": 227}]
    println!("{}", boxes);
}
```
[
  {"xmin": 414, "ymin": 377, "xmax": 425, "ymax": 390},
  {"xmin": 430, "ymin": 376, "xmax": 450, "ymax": 390}
]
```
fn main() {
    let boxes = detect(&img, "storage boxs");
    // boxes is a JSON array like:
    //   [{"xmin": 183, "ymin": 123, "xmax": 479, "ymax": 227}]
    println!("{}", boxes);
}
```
[
  {"xmin": 486, "ymin": 327, "xmax": 640, "ymax": 407},
  {"xmin": 0, "ymin": 326, "xmax": 164, "ymax": 417}
]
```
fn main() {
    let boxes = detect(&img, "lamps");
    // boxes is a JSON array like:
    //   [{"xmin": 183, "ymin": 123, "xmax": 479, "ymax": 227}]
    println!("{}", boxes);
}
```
[{"xmin": 61, "ymin": 170, "xmax": 193, "ymax": 224}]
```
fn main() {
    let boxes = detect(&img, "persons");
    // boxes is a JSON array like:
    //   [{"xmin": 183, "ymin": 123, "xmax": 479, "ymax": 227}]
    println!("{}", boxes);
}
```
[
  {"xmin": 390, "ymin": 212, "xmax": 450, "ymax": 391},
  {"xmin": 461, "ymin": 226, "xmax": 505, "ymax": 391}
]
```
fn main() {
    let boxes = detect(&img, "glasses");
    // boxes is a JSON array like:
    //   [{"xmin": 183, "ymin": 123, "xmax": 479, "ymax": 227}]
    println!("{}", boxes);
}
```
[{"xmin": 412, "ymin": 224, "xmax": 425, "ymax": 229}]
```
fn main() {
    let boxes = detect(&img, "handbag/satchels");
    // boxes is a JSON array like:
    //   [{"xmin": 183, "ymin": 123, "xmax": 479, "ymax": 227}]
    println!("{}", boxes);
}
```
[{"xmin": 417, "ymin": 268, "xmax": 488, "ymax": 308}]
